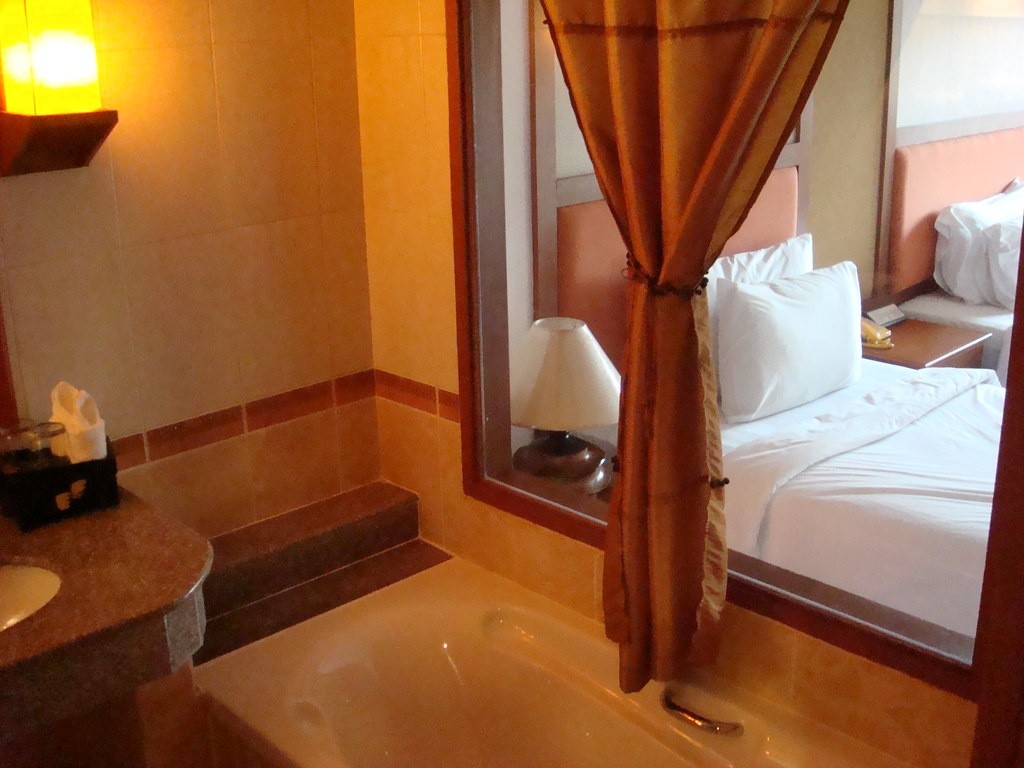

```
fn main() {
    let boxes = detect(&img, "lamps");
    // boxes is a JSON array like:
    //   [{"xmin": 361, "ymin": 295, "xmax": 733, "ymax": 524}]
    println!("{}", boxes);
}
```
[
  {"xmin": 501, "ymin": 316, "xmax": 621, "ymax": 496},
  {"xmin": 0, "ymin": 0, "xmax": 118, "ymax": 176}
]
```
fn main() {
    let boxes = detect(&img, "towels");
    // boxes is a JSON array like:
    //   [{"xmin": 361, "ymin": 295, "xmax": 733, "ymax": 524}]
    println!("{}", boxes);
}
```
[{"xmin": 48, "ymin": 381, "xmax": 106, "ymax": 464}]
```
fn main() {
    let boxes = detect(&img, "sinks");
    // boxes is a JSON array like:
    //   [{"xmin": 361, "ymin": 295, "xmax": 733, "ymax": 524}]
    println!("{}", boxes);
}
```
[{"xmin": 0, "ymin": 555, "xmax": 63, "ymax": 632}]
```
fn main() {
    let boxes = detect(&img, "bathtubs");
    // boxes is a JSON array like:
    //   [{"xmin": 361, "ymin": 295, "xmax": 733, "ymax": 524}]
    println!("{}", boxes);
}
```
[{"xmin": 143, "ymin": 557, "xmax": 910, "ymax": 768}]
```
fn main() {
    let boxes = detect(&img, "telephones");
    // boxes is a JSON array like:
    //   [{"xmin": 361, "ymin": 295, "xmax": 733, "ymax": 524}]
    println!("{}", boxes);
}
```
[{"xmin": 861, "ymin": 317, "xmax": 892, "ymax": 345}]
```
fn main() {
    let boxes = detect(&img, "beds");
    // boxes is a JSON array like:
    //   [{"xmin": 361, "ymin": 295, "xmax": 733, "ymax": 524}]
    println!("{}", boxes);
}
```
[
  {"xmin": 525, "ymin": 166, "xmax": 1006, "ymax": 640},
  {"xmin": 888, "ymin": 110, "xmax": 1024, "ymax": 388}
]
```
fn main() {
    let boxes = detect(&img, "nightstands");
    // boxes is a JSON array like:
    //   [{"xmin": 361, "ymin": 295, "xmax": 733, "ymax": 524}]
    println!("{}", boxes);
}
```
[{"xmin": 862, "ymin": 319, "xmax": 992, "ymax": 370}]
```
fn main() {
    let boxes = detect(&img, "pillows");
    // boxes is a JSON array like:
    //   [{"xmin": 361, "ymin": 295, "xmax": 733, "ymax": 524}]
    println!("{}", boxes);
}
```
[
  {"xmin": 704, "ymin": 233, "xmax": 861, "ymax": 424},
  {"xmin": 933, "ymin": 178, "xmax": 1024, "ymax": 312}
]
